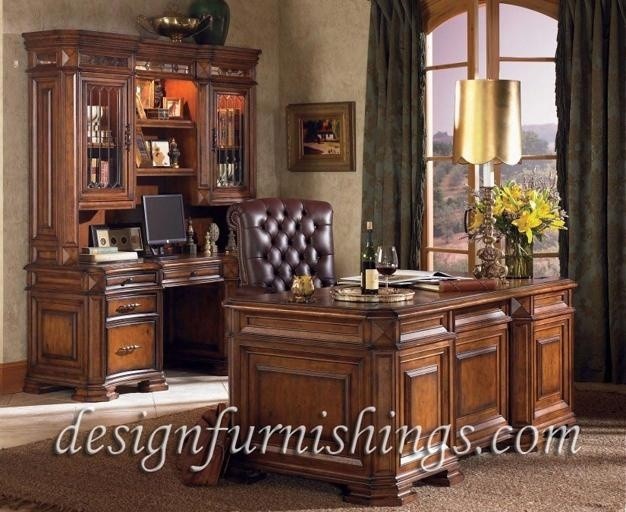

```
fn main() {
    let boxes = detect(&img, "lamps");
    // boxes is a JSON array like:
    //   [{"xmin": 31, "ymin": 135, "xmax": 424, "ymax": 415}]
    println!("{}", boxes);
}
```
[{"xmin": 451, "ymin": 79, "xmax": 522, "ymax": 279}]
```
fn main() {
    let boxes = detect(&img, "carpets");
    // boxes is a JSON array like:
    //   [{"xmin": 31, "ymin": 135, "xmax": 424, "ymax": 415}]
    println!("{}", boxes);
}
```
[{"xmin": 1, "ymin": 390, "xmax": 626, "ymax": 512}]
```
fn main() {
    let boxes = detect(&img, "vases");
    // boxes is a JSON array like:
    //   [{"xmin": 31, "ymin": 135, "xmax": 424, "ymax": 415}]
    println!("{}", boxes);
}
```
[
  {"xmin": 505, "ymin": 236, "xmax": 533, "ymax": 279},
  {"xmin": 190, "ymin": 0, "xmax": 230, "ymax": 45}
]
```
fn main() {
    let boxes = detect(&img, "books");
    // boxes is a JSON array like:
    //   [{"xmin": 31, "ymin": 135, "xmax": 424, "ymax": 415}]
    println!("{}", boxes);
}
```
[
  {"xmin": 82, "ymin": 247, "xmax": 118, "ymax": 254},
  {"xmin": 216, "ymin": 108, "xmax": 243, "ymax": 147},
  {"xmin": 89, "ymin": 157, "xmax": 110, "ymax": 188},
  {"xmin": 336, "ymin": 270, "xmax": 476, "ymax": 286},
  {"xmin": 78, "ymin": 252, "xmax": 138, "ymax": 262}
]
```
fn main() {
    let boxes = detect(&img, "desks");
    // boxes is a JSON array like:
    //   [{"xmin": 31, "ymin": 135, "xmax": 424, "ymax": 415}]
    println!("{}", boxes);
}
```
[{"xmin": 223, "ymin": 279, "xmax": 578, "ymax": 507}]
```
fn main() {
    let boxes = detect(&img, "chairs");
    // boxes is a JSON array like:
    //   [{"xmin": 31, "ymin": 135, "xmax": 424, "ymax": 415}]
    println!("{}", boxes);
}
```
[{"xmin": 227, "ymin": 198, "xmax": 337, "ymax": 295}]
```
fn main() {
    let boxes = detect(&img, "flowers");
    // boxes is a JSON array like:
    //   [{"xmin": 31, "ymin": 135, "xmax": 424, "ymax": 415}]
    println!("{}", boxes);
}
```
[{"xmin": 458, "ymin": 167, "xmax": 570, "ymax": 279}]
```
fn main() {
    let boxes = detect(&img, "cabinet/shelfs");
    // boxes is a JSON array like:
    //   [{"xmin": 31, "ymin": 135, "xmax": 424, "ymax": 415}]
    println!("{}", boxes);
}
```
[{"xmin": 20, "ymin": 28, "xmax": 262, "ymax": 403}]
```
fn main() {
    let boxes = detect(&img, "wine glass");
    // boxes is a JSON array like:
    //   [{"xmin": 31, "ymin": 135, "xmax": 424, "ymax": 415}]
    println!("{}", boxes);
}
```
[{"xmin": 376, "ymin": 245, "xmax": 399, "ymax": 289}]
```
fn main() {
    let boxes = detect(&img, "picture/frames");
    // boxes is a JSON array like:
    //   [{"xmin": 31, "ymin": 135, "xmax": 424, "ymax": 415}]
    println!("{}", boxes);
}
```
[
  {"xmin": 136, "ymin": 79, "xmax": 155, "ymax": 109},
  {"xmin": 89, "ymin": 223, "xmax": 148, "ymax": 256},
  {"xmin": 163, "ymin": 96, "xmax": 184, "ymax": 119},
  {"xmin": 145, "ymin": 140, "xmax": 173, "ymax": 167},
  {"xmin": 286, "ymin": 101, "xmax": 356, "ymax": 172}
]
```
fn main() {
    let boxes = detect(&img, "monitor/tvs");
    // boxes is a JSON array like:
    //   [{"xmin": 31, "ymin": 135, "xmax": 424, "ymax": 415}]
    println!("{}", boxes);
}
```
[{"xmin": 140, "ymin": 194, "xmax": 187, "ymax": 260}]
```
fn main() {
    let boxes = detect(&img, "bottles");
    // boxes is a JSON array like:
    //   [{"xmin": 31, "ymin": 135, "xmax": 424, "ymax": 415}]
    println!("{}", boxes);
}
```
[{"xmin": 358, "ymin": 220, "xmax": 380, "ymax": 296}]
